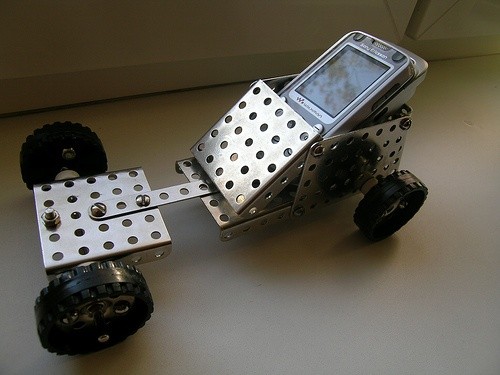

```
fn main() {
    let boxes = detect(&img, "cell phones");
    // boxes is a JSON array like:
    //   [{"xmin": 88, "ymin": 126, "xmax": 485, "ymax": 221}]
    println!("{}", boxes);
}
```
[{"xmin": 277, "ymin": 30, "xmax": 428, "ymax": 143}]
